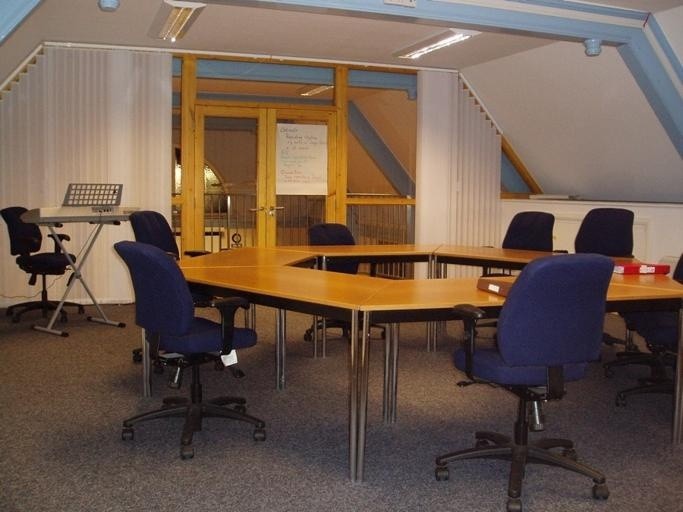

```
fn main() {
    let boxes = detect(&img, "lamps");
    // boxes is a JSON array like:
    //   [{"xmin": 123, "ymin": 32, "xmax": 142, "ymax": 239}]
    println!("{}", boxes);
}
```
[
  {"xmin": 147, "ymin": 0, "xmax": 207, "ymax": 41},
  {"xmin": 392, "ymin": 28, "xmax": 483, "ymax": 60},
  {"xmin": 295, "ymin": 85, "xmax": 334, "ymax": 96}
]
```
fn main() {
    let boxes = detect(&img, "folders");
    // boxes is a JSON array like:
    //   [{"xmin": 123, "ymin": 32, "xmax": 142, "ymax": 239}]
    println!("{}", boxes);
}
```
[
  {"xmin": 477, "ymin": 278, "xmax": 513, "ymax": 297},
  {"xmin": 612, "ymin": 261, "xmax": 670, "ymax": 274}
]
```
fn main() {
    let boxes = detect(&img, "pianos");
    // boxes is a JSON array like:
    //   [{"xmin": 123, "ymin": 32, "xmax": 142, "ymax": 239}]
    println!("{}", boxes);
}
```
[{"xmin": 21, "ymin": 209, "xmax": 140, "ymax": 224}]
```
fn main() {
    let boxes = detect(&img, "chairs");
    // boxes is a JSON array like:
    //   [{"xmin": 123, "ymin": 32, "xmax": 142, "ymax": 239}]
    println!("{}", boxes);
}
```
[
  {"xmin": 0, "ymin": 207, "xmax": 76, "ymax": 323},
  {"xmin": 114, "ymin": 241, "xmax": 266, "ymax": 459},
  {"xmin": 128, "ymin": 211, "xmax": 212, "ymax": 375},
  {"xmin": 304, "ymin": 223, "xmax": 385, "ymax": 342}
]
